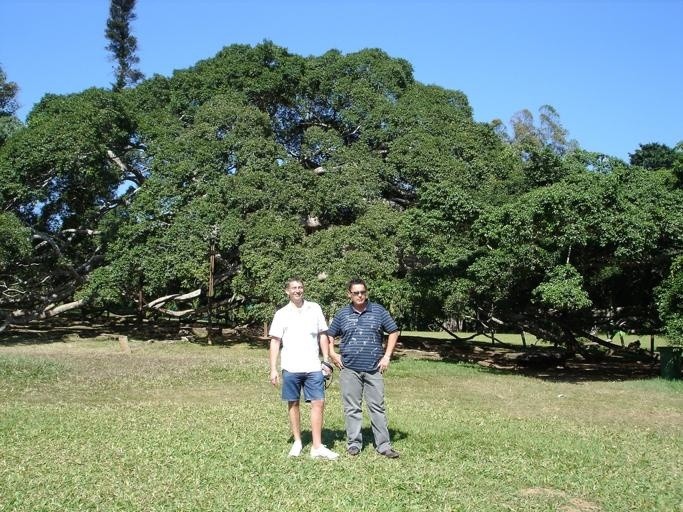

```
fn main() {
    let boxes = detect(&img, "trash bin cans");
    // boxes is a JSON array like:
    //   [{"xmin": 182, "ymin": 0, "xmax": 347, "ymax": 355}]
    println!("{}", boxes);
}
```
[{"xmin": 657, "ymin": 347, "xmax": 683, "ymax": 381}]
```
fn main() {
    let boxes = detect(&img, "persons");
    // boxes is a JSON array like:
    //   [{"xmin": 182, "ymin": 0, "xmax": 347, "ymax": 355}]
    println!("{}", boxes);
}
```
[
  {"xmin": 267, "ymin": 277, "xmax": 340, "ymax": 460},
  {"xmin": 325, "ymin": 277, "xmax": 400, "ymax": 458}
]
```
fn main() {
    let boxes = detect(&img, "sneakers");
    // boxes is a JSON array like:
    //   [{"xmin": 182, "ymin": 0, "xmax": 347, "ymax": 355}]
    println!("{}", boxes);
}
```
[
  {"xmin": 381, "ymin": 450, "xmax": 399, "ymax": 458},
  {"xmin": 349, "ymin": 447, "xmax": 359, "ymax": 455},
  {"xmin": 311, "ymin": 445, "xmax": 338, "ymax": 458},
  {"xmin": 289, "ymin": 441, "xmax": 302, "ymax": 457}
]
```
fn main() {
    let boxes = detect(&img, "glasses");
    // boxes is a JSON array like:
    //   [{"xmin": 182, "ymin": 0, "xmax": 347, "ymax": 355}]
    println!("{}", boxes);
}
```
[{"xmin": 351, "ymin": 291, "xmax": 366, "ymax": 295}]
